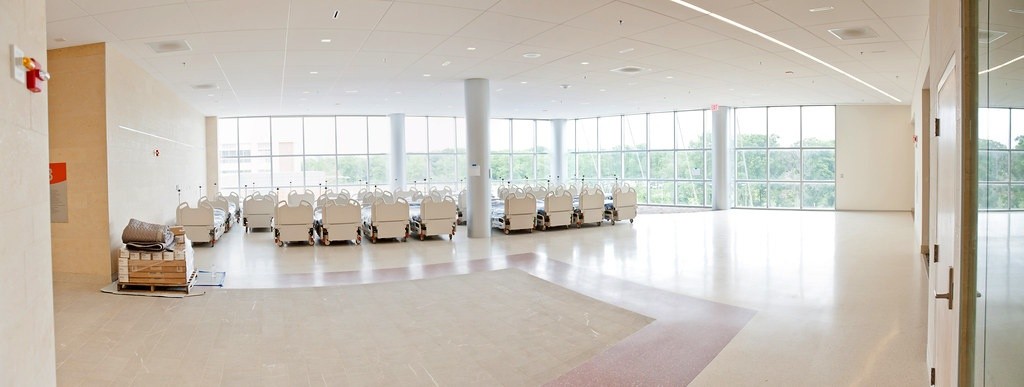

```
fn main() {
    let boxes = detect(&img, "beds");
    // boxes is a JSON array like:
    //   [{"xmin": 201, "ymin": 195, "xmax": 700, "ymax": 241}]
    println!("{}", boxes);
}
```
[
  {"xmin": 430, "ymin": 186, "xmax": 466, "ymax": 226},
  {"xmin": 396, "ymin": 184, "xmax": 422, "ymax": 201},
  {"xmin": 201, "ymin": 187, "xmax": 241, "ymax": 227},
  {"xmin": 317, "ymin": 188, "xmax": 350, "ymax": 206},
  {"xmin": 241, "ymin": 187, "xmax": 279, "ymax": 229},
  {"xmin": 358, "ymin": 185, "xmax": 394, "ymax": 207},
  {"xmin": 411, "ymin": 191, "xmax": 461, "ymax": 240},
  {"xmin": 361, "ymin": 190, "xmax": 412, "ymax": 244},
  {"xmin": 316, "ymin": 193, "xmax": 365, "ymax": 247},
  {"xmin": 287, "ymin": 187, "xmax": 316, "ymax": 206},
  {"xmin": 492, "ymin": 180, "xmax": 640, "ymax": 234},
  {"xmin": 175, "ymin": 203, "xmax": 228, "ymax": 246},
  {"xmin": 272, "ymin": 200, "xmax": 314, "ymax": 246}
]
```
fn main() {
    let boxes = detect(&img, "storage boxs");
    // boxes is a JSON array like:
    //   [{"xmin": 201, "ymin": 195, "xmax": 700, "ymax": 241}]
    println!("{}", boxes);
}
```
[{"xmin": 116, "ymin": 226, "xmax": 194, "ymax": 284}]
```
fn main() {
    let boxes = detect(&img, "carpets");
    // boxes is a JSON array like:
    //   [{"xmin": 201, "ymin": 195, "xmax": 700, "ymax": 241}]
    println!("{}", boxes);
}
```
[{"xmin": 53, "ymin": 266, "xmax": 657, "ymax": 387}]
